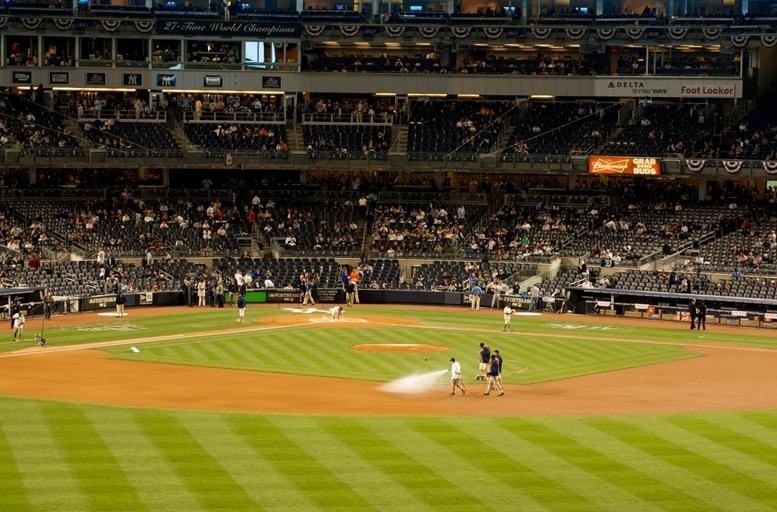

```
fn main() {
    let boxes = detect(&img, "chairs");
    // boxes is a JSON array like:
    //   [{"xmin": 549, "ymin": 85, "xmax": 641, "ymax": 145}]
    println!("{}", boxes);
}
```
[
  {"xmin": 0, "ymin": 49, "xmax": 777, "ymax": 173},
  {"xmin": 0, "ymin": 177, "xmax": 777, "ymax": 319}
]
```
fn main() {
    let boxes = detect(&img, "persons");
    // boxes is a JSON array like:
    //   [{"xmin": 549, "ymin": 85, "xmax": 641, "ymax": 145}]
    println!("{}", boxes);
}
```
[
  {"xmin": 0, "ymin": 1, "xmax": 776, "ymax": 332},
  {"xmin": 475, "ymin": 342, "xmax": 504, "ymax": 396},
  {"xmin": 449, "ymin": 359, "xmax": 465, "ymax": 396},
  {"xmin": 11, "ymin": 311, "xmax": 25, "ymax": 340}
]
